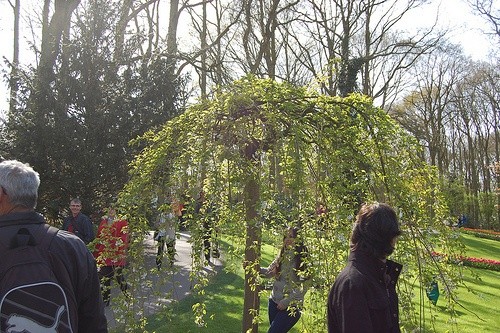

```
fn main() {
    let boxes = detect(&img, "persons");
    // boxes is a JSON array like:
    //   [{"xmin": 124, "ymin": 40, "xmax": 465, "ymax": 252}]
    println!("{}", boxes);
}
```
[
  {"xmin": 62, "ymin": 199, "xmax": 93, "ymax": 246},
  {"xmin": 0, "ymin": 159, "xmax": 107, "ymax": 333},
  {"xmin": 94, "ymin": 203, "xmax": 130, "ymax": 310},
  {"xmin": 243, "ymin": 227, "xmax": 314, "ymax": 333},
  {"xmin": 328, "ymin": 203, "xmax": 403, "ymax": 333},
  {"xmin": 155, "ymin": 201, "xmax": 175, "ymax": 272},
  {"xmin": 195, "ymin": 190, "xmax": 216, "ymax": 264},
  {"xmin": 172, "ymin": 190, "xmax": 206, "ymax": 232}
]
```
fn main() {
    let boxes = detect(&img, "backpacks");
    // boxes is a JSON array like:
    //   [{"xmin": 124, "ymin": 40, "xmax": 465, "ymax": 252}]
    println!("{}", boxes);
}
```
[{"xmin": 1, "ymin": 223, "xmax": 78, "ymax": 332}]
